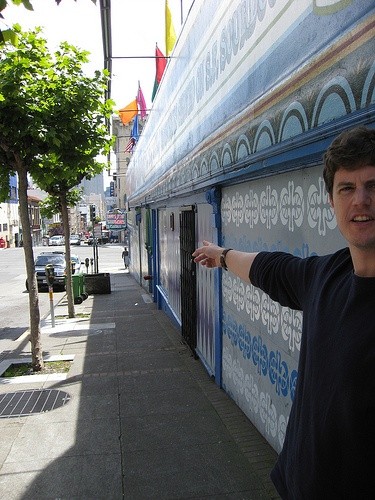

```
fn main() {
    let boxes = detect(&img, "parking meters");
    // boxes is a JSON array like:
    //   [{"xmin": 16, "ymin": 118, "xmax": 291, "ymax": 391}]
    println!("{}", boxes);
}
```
[
  {"xmin": 44, "ymin": 263, "xmax": 56, "ymax": 327},
  {"xmin": 91, "ymin": 258, "xmax": 94, "ymax": 273},
  {"xmin": 85, "ymin": 258, "xmax": 89, "ymax": 273}
]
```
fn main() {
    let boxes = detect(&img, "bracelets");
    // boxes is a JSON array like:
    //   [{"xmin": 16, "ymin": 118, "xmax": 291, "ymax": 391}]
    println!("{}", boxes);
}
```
[{"xmin": 220, "ymin": 248, "xmax": 233, "ymax": 271}]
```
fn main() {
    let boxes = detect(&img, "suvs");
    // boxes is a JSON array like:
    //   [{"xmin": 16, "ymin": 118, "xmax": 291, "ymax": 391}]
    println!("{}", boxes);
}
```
[{"xmin": 26, "ymin": 250, "xmax": 67, "ymax": 291}]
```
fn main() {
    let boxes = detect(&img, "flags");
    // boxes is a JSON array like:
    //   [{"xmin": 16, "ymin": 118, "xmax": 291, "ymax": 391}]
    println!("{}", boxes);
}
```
[{"xmin": 119, "ymin": 0, "xmax": 178, "ymax": 156}]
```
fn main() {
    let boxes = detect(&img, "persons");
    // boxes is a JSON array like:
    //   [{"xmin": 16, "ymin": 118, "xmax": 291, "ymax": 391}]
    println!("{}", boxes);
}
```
[
  {"xmin": 191, "ymin": 122, "xmax": 375, "ymax": 500},
  {"xmin": 122, "ymin": 247, "xmax": 129, "ymax": 269}
]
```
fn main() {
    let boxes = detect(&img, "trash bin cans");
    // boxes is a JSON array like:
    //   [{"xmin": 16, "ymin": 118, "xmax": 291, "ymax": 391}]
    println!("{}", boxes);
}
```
[
  {"xmin": 81, "ymin": 275, "xmax": 88, "ymax": 300},
  {"xmin": 64, "ymin": 276, "xmax": 83, "ymax": 304}
]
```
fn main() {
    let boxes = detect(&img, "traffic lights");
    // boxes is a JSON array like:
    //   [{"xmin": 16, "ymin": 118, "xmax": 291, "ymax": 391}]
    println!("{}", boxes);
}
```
[
  {"xmin": 110, "ymin": 185, "xmax": 114, "ymax": 196},
  {"xmin": 90, "ymin": 205, "xmax": 96, "ymax": 222}
]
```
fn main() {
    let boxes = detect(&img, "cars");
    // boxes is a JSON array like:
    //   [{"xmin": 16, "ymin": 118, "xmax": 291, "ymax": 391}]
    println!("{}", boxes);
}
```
[
  {"xmin": 88, "ymin": 238, "xmax": 97, "ymax": 245},
  {"xmin": 48, "ymin": 235, "xmax": 65, "ymax": 246},
  {"xmin": 71, "ymin": 235, "xmax": 81, "ymax": 245},
  {"xmin": 69, "ymin": 254, "xmax": 84, "ymax": 272}
]
